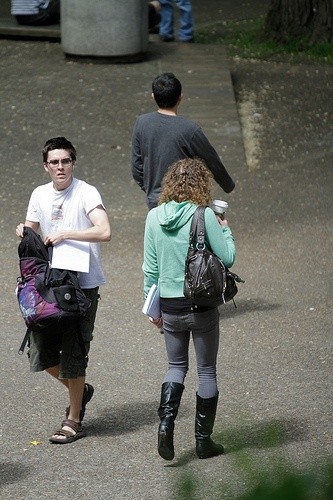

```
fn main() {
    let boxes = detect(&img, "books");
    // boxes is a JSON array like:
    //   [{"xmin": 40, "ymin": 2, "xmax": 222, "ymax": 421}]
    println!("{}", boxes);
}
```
[{"xmin": 141, "ymin": 284, "xmax": 162, "ymax": 322}]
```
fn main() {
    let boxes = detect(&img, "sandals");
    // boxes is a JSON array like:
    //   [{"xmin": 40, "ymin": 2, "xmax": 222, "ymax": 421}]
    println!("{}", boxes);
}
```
[
  {"xmin": 49, "ymin": 419, "xmax": 87, "ymax": 443},
  {"xmin": 66, "ymin": 384, "xmax": 94, "ymax": 423}
]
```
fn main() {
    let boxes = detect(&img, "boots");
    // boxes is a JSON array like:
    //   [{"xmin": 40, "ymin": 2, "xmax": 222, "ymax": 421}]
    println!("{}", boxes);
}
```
[
  {"xmin": 195, "ymin": 390, "xmax": 225, "ymax": 459},
  {"xmin": 157, "ymin": 382, "xmax": 185, "ymax": 461}
]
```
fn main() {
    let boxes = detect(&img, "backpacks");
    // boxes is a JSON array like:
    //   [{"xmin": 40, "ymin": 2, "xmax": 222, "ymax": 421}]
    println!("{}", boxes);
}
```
[{"xmin": 15, "ymin": 226, "xmax": 94, "ymax": 335}]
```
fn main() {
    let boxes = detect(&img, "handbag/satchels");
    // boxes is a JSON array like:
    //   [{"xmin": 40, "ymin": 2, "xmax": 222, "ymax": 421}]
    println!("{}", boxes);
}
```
[{"xmin": 183, "ymin": 205, "xmax": 246, "ymax": 309}]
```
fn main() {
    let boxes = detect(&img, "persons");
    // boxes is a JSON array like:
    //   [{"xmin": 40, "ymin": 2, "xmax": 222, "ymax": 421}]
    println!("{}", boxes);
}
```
[
  {"xmin": 15, "ymin": 136, "xmax": 112, "ymax": 443},
  {"xmin": 141, "ymin": 159, "xmax": 235, "ymax": 460},
  {"xmin": 131, "ymin": 72, "xmax": 235, "ymax": 212},
  {"xmin": 11, "ymin": 0, "xmax": 59, "ymax": 26},
  {"xmin": 154, "ymin": 0, "xmax": 194, "ymax": 42}
]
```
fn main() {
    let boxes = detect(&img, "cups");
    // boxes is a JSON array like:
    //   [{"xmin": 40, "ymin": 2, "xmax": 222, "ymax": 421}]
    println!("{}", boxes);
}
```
[{"xmin": 212, "ymin": 200, "xmax": 228, "ymax": 224}]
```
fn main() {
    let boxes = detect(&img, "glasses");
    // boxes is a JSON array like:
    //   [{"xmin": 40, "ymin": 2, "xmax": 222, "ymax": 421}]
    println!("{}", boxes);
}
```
[{"xmin": 45, "ymin": 158, "xmax": 75, "ymax": 167}]
